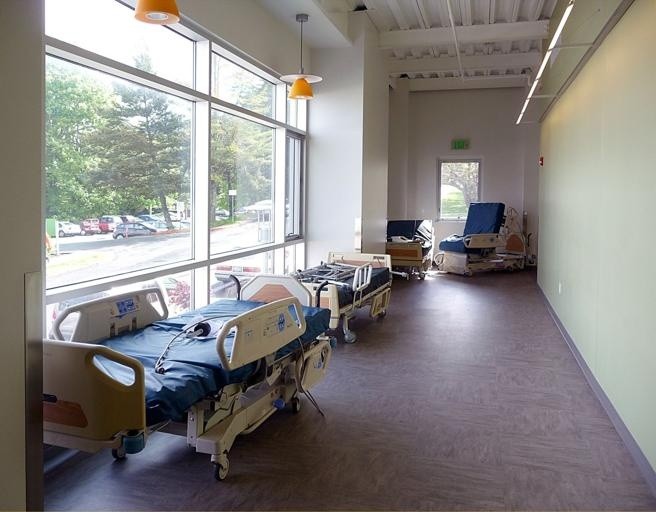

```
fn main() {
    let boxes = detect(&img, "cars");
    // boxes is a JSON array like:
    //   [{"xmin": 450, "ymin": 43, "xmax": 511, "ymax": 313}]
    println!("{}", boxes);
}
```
[
  {"xmin": 216, "ymin": 208, "xmax": 229, "ymax": 217},
  {"xmin": 59, "ymin": 211, "xmax": 179, "ymax": 239}
]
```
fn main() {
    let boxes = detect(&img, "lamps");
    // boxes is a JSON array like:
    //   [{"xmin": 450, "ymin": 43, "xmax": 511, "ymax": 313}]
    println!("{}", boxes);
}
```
[
  {"xmin": 280, "ymin": 14, "xmax": 323, "ymax": 101},
  {"xmin": 134, "ymin": 1, "xmax": 180, "ymax": 27}
]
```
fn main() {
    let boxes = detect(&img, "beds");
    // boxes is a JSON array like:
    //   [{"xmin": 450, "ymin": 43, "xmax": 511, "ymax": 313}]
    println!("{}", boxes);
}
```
[
  {"xmin": 239, "ymin": 251, "xmax": 392, "ymax": 343},
  {"xmin": 385, "ymin": 219, "xmax": 435, "ymax": 283},
  {"xmin": 45, "ymin": 275, "xmax": 334, "ymax": 478},
  {"xmin": 433, "ymin": 203, "xmax": 537, "ymax": 278}
]
